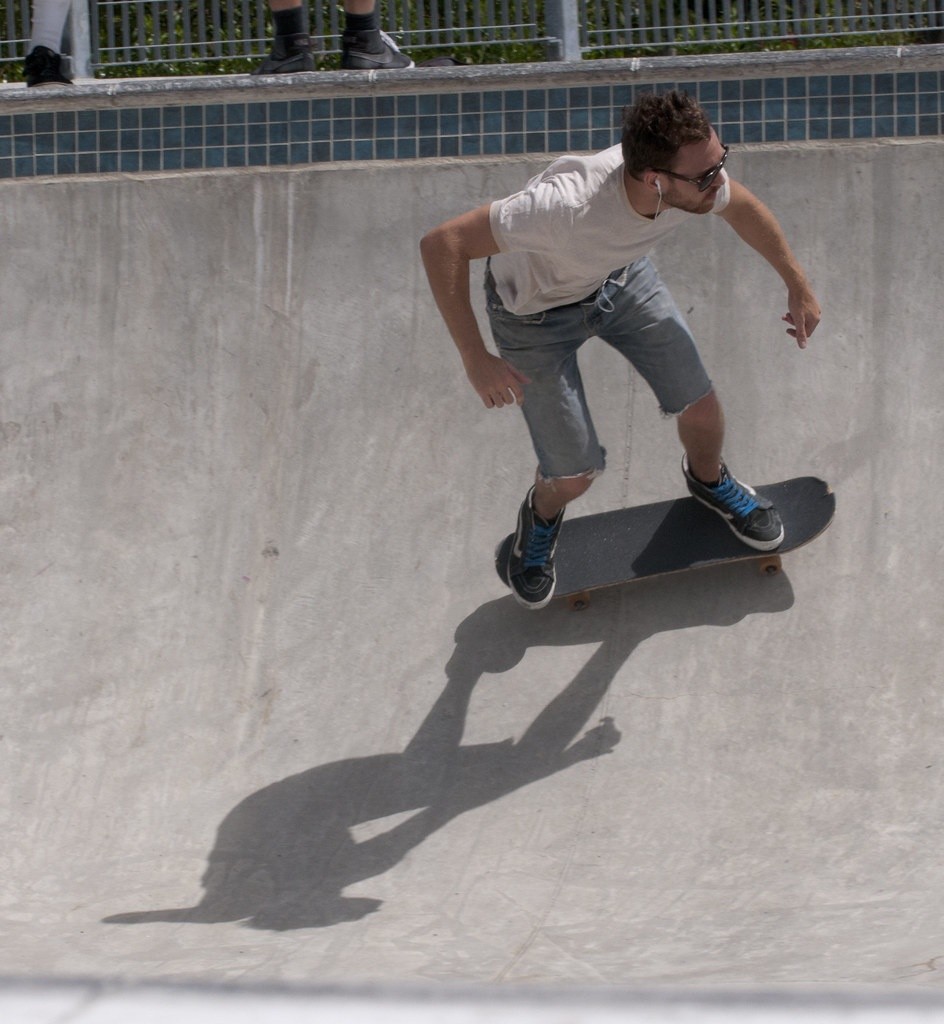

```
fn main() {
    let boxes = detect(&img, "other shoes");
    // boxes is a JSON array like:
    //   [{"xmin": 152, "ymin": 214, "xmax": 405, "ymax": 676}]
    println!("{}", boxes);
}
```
[{"xmin": 22, "ymin": 46, "xmax": 72, "ymax": 86}]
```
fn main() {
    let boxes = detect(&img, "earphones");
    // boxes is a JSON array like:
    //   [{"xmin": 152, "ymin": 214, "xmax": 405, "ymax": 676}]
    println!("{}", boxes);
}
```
[{"xmin": 655, "ymin": 179, "xmax": 662, "ymax": 197}]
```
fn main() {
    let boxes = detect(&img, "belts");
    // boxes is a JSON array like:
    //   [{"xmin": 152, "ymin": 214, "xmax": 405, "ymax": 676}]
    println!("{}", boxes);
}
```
[{"xmin": 488, "ymin": 267, "xmax": 624, "ymax": 306}]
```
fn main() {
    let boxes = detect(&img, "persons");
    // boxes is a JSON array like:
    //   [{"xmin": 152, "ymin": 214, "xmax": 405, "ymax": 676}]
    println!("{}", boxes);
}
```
[
  {"xmin": 419, "ymin": 93, "xmax": 821, "ymax": 611},
  {"xmin": 249, "ymin": 0, "xmax": 414, "ymax": 76},
  {"xmin": 22, "ymin": 0, "xmax": 73, "ymax": 87}
]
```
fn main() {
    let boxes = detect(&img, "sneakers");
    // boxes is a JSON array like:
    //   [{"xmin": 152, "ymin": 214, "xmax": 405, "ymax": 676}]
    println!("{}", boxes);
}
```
[
  {"xmin": 506, "ymin": 484, "xmax": 566, "ymax": 610},
  {"xmin": 682, "ymin": 452, "xmax": 784, "ymax": 551},
  {"xmin": 342, "ymin": 29, "xmax": 415, "ymax": 68},
  {"xmin": 250, "ymin": 34, "xmax": 316, "ymax": 74}
]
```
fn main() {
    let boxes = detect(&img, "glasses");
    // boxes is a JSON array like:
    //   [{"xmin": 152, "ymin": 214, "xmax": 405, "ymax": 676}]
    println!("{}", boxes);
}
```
[{"xmin": 651, "ymin": 143, "xmax": 730, "ymax": 191}]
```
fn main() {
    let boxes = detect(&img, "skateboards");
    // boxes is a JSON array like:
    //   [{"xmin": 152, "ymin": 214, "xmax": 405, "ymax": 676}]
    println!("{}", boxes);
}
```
[{"xmin": 496, "ymin": 474, "xmax": 838, "ymax": 610}]
